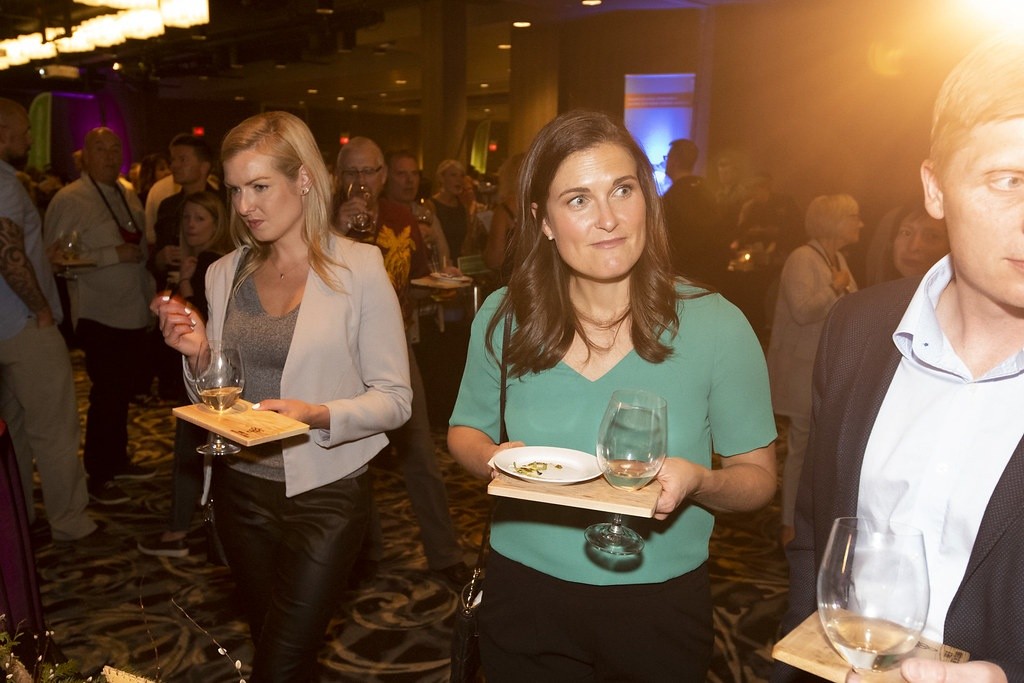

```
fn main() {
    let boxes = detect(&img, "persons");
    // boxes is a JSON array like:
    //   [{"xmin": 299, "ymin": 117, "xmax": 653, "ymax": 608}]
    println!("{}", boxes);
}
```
[
  {"xmin": 149, "ymin": 111, "xmax": 414, "ymax": 683},
  {"xmin": 0, "ymin": 98, "xmax": 121, "ymax": 551},
  {"xmin": 45, "ymin": 127, "xmax": 157, "ymax": 504},
  {"xmin": 766, "ymin": 196, "xmax": 865, "ymax": 547},
  {"xmin": 136, "ymin": 190, "xmax": 232, "ymax": 557},
  {"xmin": 776, "ymin": 35, "xmax": 1024, "ymax": 683},
  {"xmin": 657, "ymin": 138, "xmax": 805, "ymax": 310},
  {"xmin": 128, "ymin": 132, "xmax": 221, "ymax": 290},
  {"xmin": 448, "ymin": 109, "xmax": 778, "ymax": 683},
  {"xmin": 44, "ymin": 167, "xmax": 67, "ymax": 206},
  {"xmin": 877, "ymin": 200, "xmax": 950, "ymax": 280},
  {"xmin": 381, "ymin": 150, "xmax": 524, "ymax": 275},
  {"xmin": 332, "ymin": 137, "xmax": 472, "ymax": 589}
]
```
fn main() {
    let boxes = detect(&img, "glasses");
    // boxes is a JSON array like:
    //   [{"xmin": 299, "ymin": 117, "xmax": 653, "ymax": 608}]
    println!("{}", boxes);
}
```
[
  {"xmin": 847, "ymin": 215, "xmax": 862, "ymax": 225},
  {"xmin": 664, "ymin": 156, "xmax": 670, "ymax": 161},
  {"xmin": 339, "ymin": 167, "xmax": 381, "ymax": 181}
]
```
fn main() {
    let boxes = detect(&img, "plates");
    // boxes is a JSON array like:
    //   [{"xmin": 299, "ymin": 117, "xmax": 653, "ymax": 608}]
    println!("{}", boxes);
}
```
[
  {"xmin": 431, "ymin": 272, "xmax": 471, "ymax": 283},
  {"xmin": 493, "ymin": 444, "xmax": 608, "ymax": 486}
]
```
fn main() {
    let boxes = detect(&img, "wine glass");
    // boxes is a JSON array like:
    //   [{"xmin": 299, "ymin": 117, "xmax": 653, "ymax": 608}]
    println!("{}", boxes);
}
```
[
  {"xmin": 347, "ymin": 181, "xmax": 375, "ymax": 232},
  {"xmin": 120, "ymin": 214, "xmax": 144, "ymax": 263},
  {"xmin": 195, "ymin": 339, "xmax": 241, "ymax": 456},
  {"xmin": 581, "ymin": 387, "xmax": 668, "ymax": 555},
  {"xmin": 410, "ymin": 197, "xmax": 433, "ymax": 242},
  {"xmin": 817, "ymin": 516, "xmax": 930, "ymax": 683},
  {"xmin": 57, "ymin": 230, "xmax": 81, "ymax": 280}
]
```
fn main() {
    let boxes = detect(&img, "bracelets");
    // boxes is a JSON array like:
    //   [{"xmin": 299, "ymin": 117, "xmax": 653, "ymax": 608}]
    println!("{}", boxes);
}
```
[{"xmin": 179, "ymin": 278, "xmax": 188, "ymax": 284}]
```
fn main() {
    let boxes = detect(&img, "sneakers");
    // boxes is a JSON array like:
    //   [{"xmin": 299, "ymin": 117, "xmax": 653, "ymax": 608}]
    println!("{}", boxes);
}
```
[
  {"xmin": 87, "ymin": 476, "xmax": 130, "ymax": 505},
  {"xmin": 431, "ymin": 561, "xmax": 472, "ymax": 594},
  {"xmin": 53, "ymin": 529, "xmax": 123, "ymax": 563},
  {"xmin": 113, "ymin": 465, "xmax": 157, "ymax": 480},
  {"xmin": 137, "ymin": 535, "xmax": 190, "ymax": 558},
  {"xmin": 352, "ymin": 560, "xmax": 377, "ymax": 588}
]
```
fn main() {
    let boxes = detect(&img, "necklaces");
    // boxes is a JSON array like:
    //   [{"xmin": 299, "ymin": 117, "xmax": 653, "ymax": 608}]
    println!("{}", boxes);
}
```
[{"xmin": 280, "ymin": 274, "xmax": 285, "ymax": 279}]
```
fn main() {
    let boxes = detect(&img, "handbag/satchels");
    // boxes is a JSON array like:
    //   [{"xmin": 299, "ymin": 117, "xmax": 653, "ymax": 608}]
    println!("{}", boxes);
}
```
[{"xmin": 451, "ymin": 576, "xmax": 486, "ymax": 683}]
